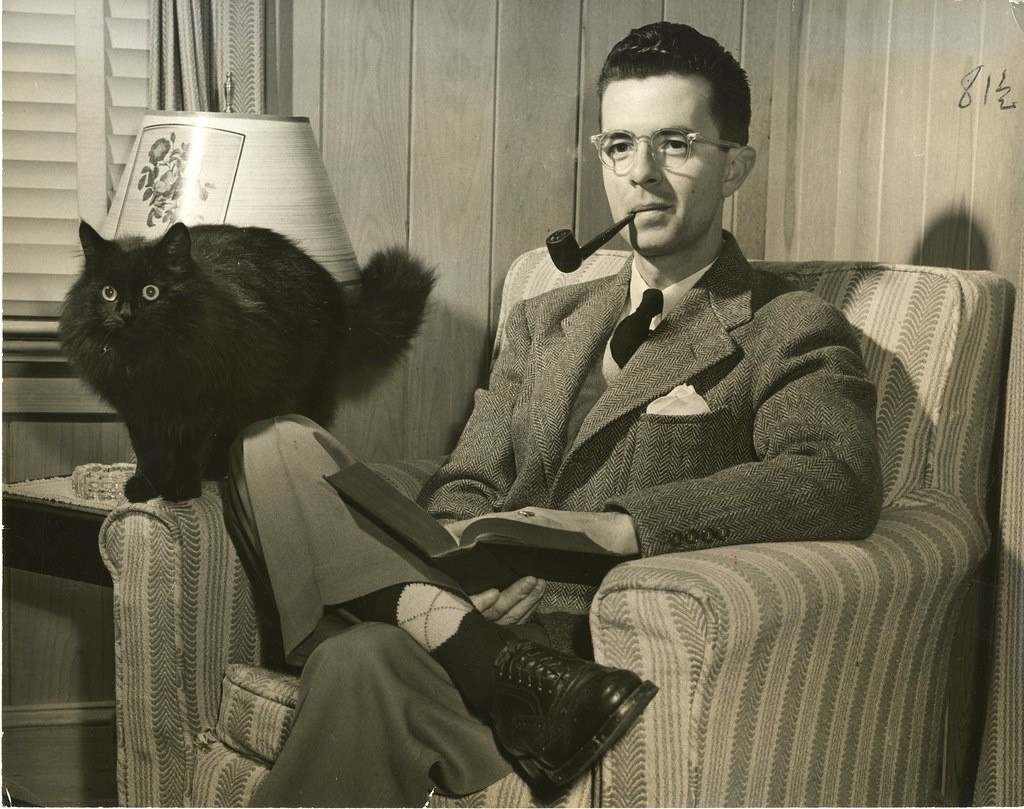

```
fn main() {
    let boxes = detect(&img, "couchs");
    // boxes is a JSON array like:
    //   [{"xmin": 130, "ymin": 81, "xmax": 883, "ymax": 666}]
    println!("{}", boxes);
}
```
[{"xmin": 99, "ymin": 247, "xmax": 1015, "ymax": 807}]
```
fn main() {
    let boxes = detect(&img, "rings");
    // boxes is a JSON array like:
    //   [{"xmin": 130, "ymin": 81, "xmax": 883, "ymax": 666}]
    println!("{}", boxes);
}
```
[{"xmin": 518, "ymin": 510, "xmax": 536, "ymax": 518}]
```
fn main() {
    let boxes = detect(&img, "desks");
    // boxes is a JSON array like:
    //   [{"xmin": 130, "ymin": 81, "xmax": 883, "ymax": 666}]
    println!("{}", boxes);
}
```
[{"xmin": 2, "ymin": 475, "xmax": 113, "ymax": 589}]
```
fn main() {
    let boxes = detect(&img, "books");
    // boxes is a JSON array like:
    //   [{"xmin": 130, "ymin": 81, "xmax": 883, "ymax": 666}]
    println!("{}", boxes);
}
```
[{"xmin": 320, "ymin": 460, "xmax": 623, "ymax": 596}]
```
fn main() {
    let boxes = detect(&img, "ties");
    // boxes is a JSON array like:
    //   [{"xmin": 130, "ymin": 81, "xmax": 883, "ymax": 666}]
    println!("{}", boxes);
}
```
[{"xmin": 610, "ymin": 288, "xmax": 664, "ymax": 369}]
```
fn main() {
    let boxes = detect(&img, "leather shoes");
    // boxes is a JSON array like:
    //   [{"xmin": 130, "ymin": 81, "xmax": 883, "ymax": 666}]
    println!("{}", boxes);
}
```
[{"xmin": 490, "ymin": 642, "xmax": 659, "ymax": 801}]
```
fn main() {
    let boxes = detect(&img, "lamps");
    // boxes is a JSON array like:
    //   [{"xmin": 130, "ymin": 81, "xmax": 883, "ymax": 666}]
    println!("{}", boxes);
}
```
[{"xmin": 98, "ymin": 71, "xmax": 362, "ymax": 289}]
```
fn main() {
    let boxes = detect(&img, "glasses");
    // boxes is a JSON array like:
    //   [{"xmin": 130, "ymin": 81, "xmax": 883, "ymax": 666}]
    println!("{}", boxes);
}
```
[{"xmin": 589, "ymin": 128, "xmax": 747, "ymax": 169}]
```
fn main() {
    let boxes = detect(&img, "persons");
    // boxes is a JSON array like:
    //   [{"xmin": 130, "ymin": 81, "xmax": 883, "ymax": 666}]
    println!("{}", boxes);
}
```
[{"xmin": 221, "ymin": 22, "xmax": 884, "ymax": 808}]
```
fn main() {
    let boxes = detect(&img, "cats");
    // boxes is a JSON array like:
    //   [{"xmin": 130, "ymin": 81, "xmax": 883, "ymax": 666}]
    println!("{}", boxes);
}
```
[{"xmin": 59, "ymin": 220, "xmax": 443, "ymax": 503}]
